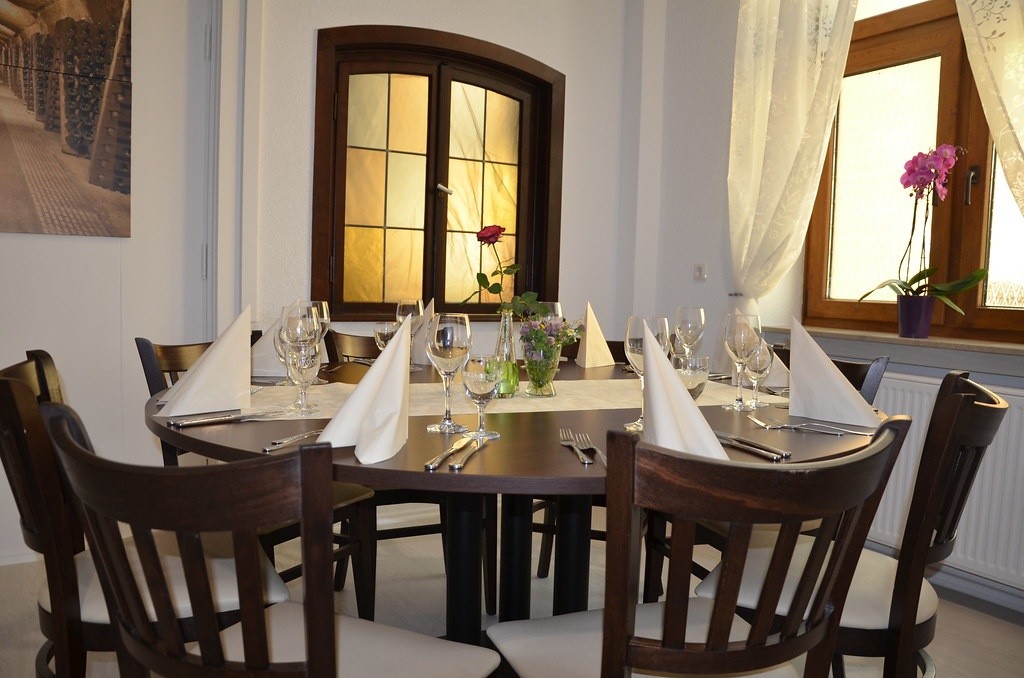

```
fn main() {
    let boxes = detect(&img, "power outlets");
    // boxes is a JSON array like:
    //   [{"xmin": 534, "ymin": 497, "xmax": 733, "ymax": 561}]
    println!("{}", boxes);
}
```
[{"xmin": 692, "ymin": 263, "xmax": 706, "ymax": 281}]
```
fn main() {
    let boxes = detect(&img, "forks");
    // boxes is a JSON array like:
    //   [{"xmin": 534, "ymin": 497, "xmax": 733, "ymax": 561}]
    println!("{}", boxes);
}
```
[
  {"xmin": 575, "ymin": 433, "xmax": 607, "ymax": 466},
  {"xmin": 768, "ymin": 416, "xmax": 874, "ymax": 436},
  {"xmin": 747, "ymin": 415, "xmax": 844, "ymax": 437},
  {"xmin": 621, "ymin": 365, "xmax": 634, "ymax": 371},
  {"xmin": 560, "ymin": 427, "xmax": 592, "ymax": 463},
  {"xmin": 767, "ymin": 385, "xmax": 788, "ymax": 394}
]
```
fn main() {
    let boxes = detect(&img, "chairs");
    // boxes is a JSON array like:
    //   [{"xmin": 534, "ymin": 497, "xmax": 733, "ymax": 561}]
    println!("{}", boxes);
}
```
[
  {"xmin": 482, "ymin": 414, "xmax": 914, "ymax": 678},
  {"xmin": 528, "ymin": 334, "xmax": 676, "ymax": 580},
  {"xmin": 693, "ymin": 367, "xmax": 1010, "ymax": 678},
  {"xmin": 38, "ymin": 401, "xmax": 501, "ymax": 678},
  {"xmin": 643, "ymin": 342, "xmax": 872, "ymax": 604},
  {"xmin": 0, "ymin": 348, "xmax": 290, "ymax": 678},
  {"xmin": 324, "ymin": 327, "xmax": 441, "ymax": 593},
  {"xmin": 134, "ymin": 331, "xmax": 380, "ymax": 623}
]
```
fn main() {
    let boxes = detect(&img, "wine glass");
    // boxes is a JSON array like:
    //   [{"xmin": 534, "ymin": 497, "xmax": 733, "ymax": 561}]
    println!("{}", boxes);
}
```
[
  {"xmin": 744, "ymin": 344, "xmax": 773, "ymax": 408},
  {"xmin": 395, "ymin": 299, "xmax": 423, "ymax": 371},
  {"xmin": 721, "ymin": 314, "xmax": 762, "ymax": 412},
  {"xmin": 425, "ymin": 313, "xmax": 468, "ymax": 433},
  {"xmin": 462, "ymin": 356, "xmax": 500, "ymax": 439},
  {"xmin": 624, "ymin": 315, "xmax": 670, "ymax": 431},
  {"xmin": 669, "ymin": 308, "xmax": 706, "ymax": 368},
  {"xmin": 280, "ymin": 342, "xmax": 319, "ymax": 416},
  {"xmin": 274, "ymin": 301, "xmax": 329, "ymax": 385}
]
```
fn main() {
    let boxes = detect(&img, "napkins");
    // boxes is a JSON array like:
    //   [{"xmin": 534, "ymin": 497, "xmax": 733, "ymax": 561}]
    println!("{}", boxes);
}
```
[
  {"xmin": 575, "ymin": 301, "xmax": 616, "ymax": 368},
  {"xmin": 315, "ymin": 312, "xmax": 412, "ymax": 467},
  {"xmin": 410, "ymin": 298, "xmax": 441, "ymax": 366},
  {"xmin": 250, "ymin": 304, "xmax": 330, "ymax": 377},
  {"xmin": 153, "ymin": 302, "xmax": 253, "ymax": 418},
  {"xmin": 789, "ymin": 314, "xmax": 882, "ymax": 430},
  {"xmin": 642, "ymin": 318, "xmax": 731, "ymax": 460},
  {"xmin": 731, "ymin": 308, "xmax": 791, "ymax": 393}
]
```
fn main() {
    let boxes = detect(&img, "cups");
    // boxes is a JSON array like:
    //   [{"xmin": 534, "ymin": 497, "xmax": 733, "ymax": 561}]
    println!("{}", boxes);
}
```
[
  {"xmin": 538, "ymin": 301, "xmax": 563, "ymax": 323},
  {"xmin": 670, "ymin": 355, "xmax": 709, "ymax": 401},
  {"xmin": 374, "ymin": 322, "xmax": 399, "ymax": 351}
]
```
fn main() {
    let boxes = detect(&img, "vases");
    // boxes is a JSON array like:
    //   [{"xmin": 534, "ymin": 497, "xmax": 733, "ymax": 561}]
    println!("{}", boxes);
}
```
[
  {"xmin": 898, "ymin": 295, "xmax": 932, "ymax": 340},
  {"xmin": 523, "ymin": 343, "xmax": 562, "ymax": 398},
  {"xmin": 486, "ymin": 310, "xmax": 519, "ymax": 398}
]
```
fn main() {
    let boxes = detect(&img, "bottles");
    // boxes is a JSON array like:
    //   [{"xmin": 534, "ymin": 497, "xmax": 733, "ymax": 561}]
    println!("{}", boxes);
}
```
[{"xmin": 491, "ymin": 308, "xmax": 519, "ymax": 399}]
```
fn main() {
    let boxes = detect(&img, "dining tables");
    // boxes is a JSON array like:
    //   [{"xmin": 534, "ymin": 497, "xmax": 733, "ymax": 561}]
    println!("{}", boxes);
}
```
[{"xmin": 147, "ymin": 356, "xmax": 889, "ymax": 645}]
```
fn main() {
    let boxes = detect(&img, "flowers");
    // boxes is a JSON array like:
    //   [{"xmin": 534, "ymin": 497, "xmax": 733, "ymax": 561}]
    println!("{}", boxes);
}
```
[
  {"xmin": 517, "ymin": 310, "xmax": 585, "ymax": 348},
  {"xmin": 458, "ymin": 225, "xmax": 550, "ymax": 319},
  {"xmin": 855, "ymin": 143, "xmax": 988, "ymax": 319}
]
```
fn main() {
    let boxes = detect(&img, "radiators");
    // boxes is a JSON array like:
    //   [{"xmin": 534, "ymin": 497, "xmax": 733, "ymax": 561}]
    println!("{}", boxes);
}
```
[{"xmin": 864, "ymin": 360, "xmax": 1024, "ymax": 616}]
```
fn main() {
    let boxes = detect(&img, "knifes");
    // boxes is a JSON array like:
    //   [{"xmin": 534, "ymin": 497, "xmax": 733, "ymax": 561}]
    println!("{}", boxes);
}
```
[
  {"xmin": 448, "ymin": 437, "xmax": 489, "ymax": 469},
  {"xmin": 716, "ymin": 437, "xmax": 781, "ymax": 460},
  {"xmin": 707, "ymin": 373, "xmax": 732, "ymax": 379},
  {"xmin": 424, "ymin": 437, "xmax": 471, "ymax": 469},
  {"xmin": 166, "ymin": 411, "xmax": 285, "ymax": 428},
  {"xmin": 715, "ymin": 430, "xmax": 791, "ymax": 458}
]
